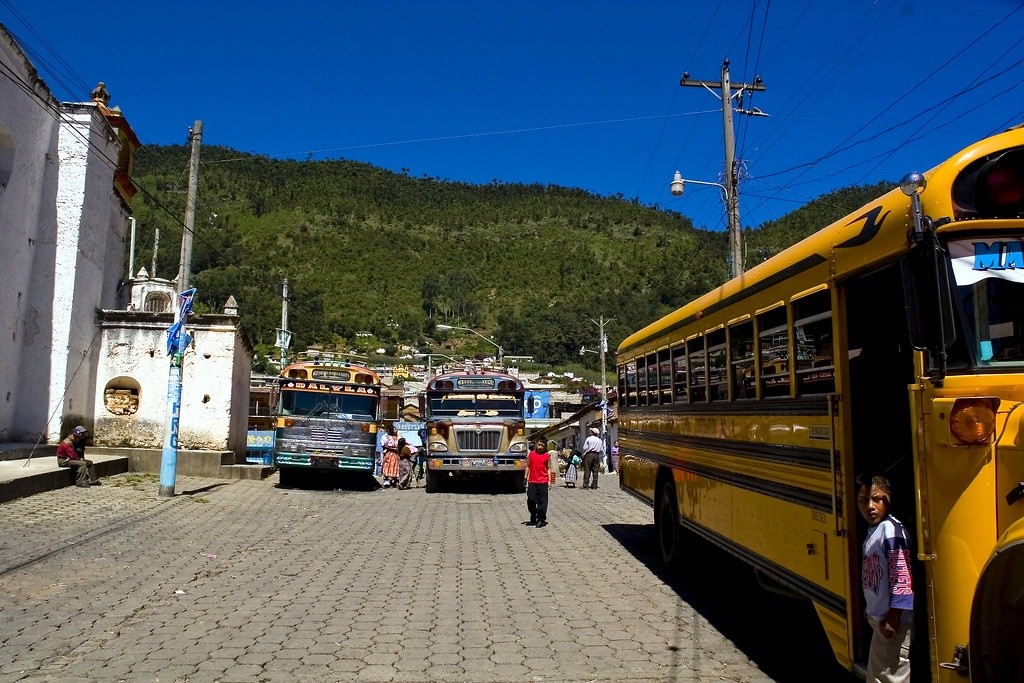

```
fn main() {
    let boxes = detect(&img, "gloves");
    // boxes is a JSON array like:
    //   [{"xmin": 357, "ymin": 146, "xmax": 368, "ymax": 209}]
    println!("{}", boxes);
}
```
[{"xmin": 522, "ymin": 478, "xmax": 527, "ymax": 486}]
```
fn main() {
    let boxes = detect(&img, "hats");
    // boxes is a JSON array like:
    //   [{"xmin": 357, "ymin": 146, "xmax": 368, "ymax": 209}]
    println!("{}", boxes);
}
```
[
  {"xmin": 590, "ymin": 428, "xmax": 600, "ymax": 435},
  {"xmin": 71, "ymin": 428, "xmax": 82, "ymax": 434},
  {"xmin": 75, "ymin": 426, "xmax": 87, "ymax": 433}
]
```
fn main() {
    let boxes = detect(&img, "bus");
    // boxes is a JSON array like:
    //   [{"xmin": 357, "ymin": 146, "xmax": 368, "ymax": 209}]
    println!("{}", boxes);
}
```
[
  {"xmin": 268, "ymin": 361, "xmax": 389, "ymax": 490},
  {"xmin": 418, "ymin": 368, "xmax": 534, "ymax": 493},
  {"xmin": 615, "ymin": 123, "xmax": 1024, "ymax": 683}
]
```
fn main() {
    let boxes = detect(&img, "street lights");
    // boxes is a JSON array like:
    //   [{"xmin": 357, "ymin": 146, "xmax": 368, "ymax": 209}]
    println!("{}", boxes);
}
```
[
  {"xmin": 669, "ymin": 170, "xmax": 743, "ymax": 280},
  {"xmin": 579, "ymin": 345, "xmax": 609, "ymax": 474},
  {"xmin": 435, "ymin": 324, "xmax": 505, "ymax": 371}
]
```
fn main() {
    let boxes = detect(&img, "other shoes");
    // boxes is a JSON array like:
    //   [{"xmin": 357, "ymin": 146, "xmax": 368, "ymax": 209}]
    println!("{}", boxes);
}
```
[
  {"xmin": 398, "ymin": 484, "xmax": 407, "ymax": 490},
  {"xmin": 88, "ymin": 479, "xmax": 102, "ymax": 485},
  {"xmin": 76, "ymin": 482, "xmax": 91, "ymax": 488},
  {"xmin": 590, "ymin": 485, "xmax": 597, "ymax": 489},
  {"xmin": 383, "ymin": 480, "xmax": 390, "ymax": 488},
  {"xmin": 579, "ymin": 485, "xmax": 589, "ymax": 490},
  {"xmin": 530, "ymin": 511, "xmax": 536, "ymax": 524},
  {"xmin": 536, "ymin": 520, "xmax": 546, "ymax": 527}
]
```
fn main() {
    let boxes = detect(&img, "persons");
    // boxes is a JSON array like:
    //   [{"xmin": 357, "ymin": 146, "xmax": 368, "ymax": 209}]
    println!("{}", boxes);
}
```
[
  {"xmin": 380, "ymin": 425, "xmax": 418, "ymax": 489},
  {"xmin": 57, "ymin": 426, "xmax": 102, "ymax": 487},
  {"xmin": 857, "ymin": 475, "xmax": 916, "ymax": 683},
  {"xmin": 579, "ymin": 428, "xmax": 603, "ymax": 490},
  {"xmin": 522, "ymin": 436, "xmax": 552, "ymax": 528},
  {"xmin": 557, "ymin": 446, "xmax": 582, "ymax": 488},
  {"xmin": 611, "ymin": 440, "xmax": 619, "ymax": 474}
]
